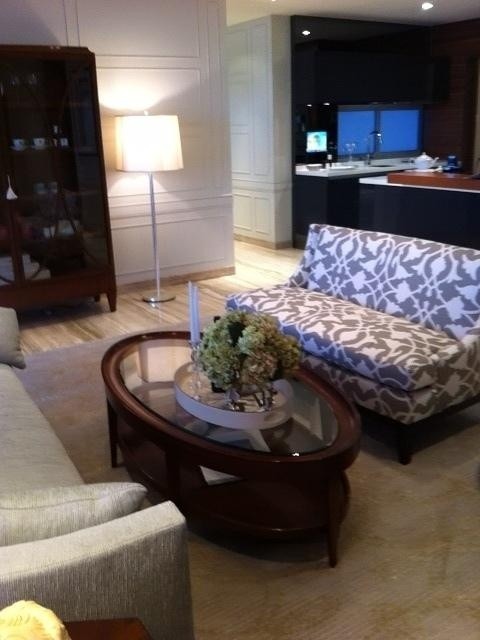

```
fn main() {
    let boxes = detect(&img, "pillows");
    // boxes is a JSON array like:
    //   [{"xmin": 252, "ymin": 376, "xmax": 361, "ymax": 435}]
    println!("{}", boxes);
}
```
[
  {"xmin": 0, "ymin": 479, "xmax": 148, "ymax": 546},
  {"xmin": 0, "ymin": 307, "xmax": 27, "ymax": 369}
]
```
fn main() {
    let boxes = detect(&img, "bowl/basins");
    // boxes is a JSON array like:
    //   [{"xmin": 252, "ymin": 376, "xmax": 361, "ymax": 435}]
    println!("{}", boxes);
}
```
[{"xmin": 306, "ymin": 164, "xmax": 322, "ymax": 171}]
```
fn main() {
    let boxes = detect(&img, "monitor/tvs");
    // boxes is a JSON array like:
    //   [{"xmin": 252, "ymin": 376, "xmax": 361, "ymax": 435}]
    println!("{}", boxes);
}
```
[
  {"xmin": 378, "ymin": 103, "xmax": 424, "ymax": 158},
  {"xmin": 335, "ymin": 103, "xmax": 378, "ymax": 162},
  {"xmin": 305, "ymin": 129, "xmax": 329, "ymax": 155}
]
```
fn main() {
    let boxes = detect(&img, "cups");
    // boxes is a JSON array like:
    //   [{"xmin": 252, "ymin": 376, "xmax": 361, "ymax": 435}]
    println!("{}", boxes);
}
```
[
  {"xmin": 13, "ymin": 139, "xmax": 25, "ymax": 146},
  {"xmin": 34, "ymin": 138, "xmax": 45, "ymax": 145}
]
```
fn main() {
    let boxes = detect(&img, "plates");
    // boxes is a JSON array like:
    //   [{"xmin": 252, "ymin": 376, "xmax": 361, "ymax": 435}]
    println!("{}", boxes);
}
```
[
  {"xmin": 10, "ymin": 146, "xmax": 29, "ymax": 152},
  {"xmin": 30, "ymin": 145, "xmax": 49, "ymax": 150},
  {"xmin": 330, "ymin": 166, "xmax": 352, "ymax": 170}
]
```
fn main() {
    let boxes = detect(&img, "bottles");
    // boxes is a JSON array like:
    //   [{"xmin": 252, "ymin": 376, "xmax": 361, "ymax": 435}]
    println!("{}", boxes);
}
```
[
  {"xmin": 52, "ymin": 125, "xmax": 60, "ymax": 149},
  {"xmin": 327, "ymin": 141, "xmax": 338, "ymax": 166}
]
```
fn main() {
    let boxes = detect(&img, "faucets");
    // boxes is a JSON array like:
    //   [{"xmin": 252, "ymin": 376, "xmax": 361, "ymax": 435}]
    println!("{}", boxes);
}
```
[{"xmin": 365, "ymin": 128, "xmax": 383, "ymax": 163}]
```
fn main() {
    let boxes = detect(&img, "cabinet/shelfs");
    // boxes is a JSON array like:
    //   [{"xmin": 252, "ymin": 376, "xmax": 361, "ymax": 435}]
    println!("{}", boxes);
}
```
[{"xmin": 0, "ymin": 43, "xmax": 116, "ymax": 314}]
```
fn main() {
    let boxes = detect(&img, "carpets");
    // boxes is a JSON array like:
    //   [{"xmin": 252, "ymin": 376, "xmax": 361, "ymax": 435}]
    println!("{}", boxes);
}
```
[{"xmin": 13, "ymin": 316, "xmax": 480, "ymax": 640}]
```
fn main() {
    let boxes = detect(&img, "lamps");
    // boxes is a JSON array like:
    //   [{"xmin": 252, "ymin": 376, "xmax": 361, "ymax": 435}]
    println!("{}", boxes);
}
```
[{"xmin": 115, "ymin": 115, "xmax": 184, "ymax": 303}]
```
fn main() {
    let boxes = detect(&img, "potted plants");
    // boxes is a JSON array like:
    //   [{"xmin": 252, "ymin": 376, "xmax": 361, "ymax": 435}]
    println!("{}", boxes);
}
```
[{"xmin": 192, "ymin": 310, "xmax": 304, "ymax": 414}]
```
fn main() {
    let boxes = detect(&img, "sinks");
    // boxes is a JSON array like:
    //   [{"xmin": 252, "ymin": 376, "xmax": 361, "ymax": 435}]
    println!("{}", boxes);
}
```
[{"xmin": 367, "ymin": 162, "xmax": 397, "ymax": 168}]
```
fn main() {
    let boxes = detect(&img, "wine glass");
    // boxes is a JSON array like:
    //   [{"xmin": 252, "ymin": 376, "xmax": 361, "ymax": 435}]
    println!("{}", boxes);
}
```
[{"xmin": 345, "ymin": 143, "xmax": 356, "ymax": 165}]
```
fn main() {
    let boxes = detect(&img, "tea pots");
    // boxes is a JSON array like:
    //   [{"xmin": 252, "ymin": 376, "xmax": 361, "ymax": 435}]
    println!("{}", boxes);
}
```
[{"xmin": 409, "ymin": 152, "xmax": 439, "ymax": 170}]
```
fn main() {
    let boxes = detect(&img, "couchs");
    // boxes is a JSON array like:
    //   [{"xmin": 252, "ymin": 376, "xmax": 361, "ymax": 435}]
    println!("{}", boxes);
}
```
[
  {"xmin": 226, "ymin": 224, "xmax": 480, "ymax": 465},
  {"xmin": 0, "ymin": 307, "xmax": 196, "ymax": 640}
]
